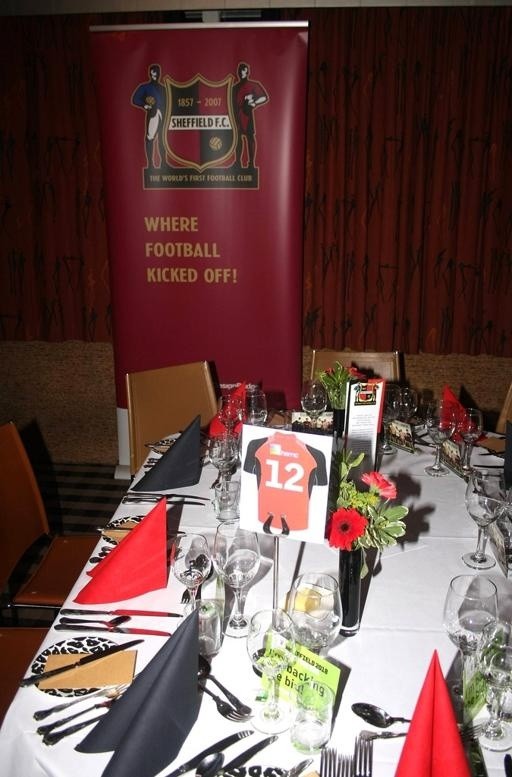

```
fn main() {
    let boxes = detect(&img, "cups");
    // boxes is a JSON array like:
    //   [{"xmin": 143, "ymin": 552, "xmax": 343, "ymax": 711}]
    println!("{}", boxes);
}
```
[
  {"xmin": 184, "ymin": 597, "xmax": 222, "ymax": 657},
  {"xmin": 243, "ymin": 389, "xmax": 269, "ymax": 426},
  {"xmin": 288, "ymin": 682, "xmax": 337, "ymax": 752},
  {"xmin": 285, "ymin": 570, "xmax": 346, "ymax": 653}
]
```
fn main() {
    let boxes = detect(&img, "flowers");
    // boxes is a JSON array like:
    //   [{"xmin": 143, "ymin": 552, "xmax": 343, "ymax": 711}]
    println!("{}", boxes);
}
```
[
  {"xmin": 321, "ymin": 445, "xmax": 410, "ymax": 579},
  {"xmin": 312, "ymin": 364, "xmax": 367, "ymax": 408}
]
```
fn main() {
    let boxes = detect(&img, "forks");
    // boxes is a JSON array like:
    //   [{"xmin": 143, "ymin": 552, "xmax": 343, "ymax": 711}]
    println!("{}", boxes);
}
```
[
  {"xmin": 33, "ymin": 679, "xmax": 125, "ymax": 746},
  {"xmin": 358, "ymin": 723, "xmax": 488, "ymax": 743},
  {"xmin": 195, "ymin": 681, "xmax": 251, "ymax": 727},
  {"xmin": 143, "ymin": 457, "xmax": 211, "ymax": 472},
  {"xmin": 318, "ymin": 733, "xmax": 376, "ymax": 777}
]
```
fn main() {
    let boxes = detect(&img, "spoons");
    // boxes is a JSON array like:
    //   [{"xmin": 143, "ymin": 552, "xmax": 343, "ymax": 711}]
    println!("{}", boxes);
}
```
[
  {"xmin": 195, "ymin": 652, "xmax": 252, "ymax": 715},
  {"xmin": 56, "ymin": 614, "xmax": 134, "ymax": 630},
  {"xmin": 350, "ymin": 698, "xmax": 411, "ymax": 727},
  {"xmin": 194, "ymin": 750, "xmax": 224, "ymax": 776}
]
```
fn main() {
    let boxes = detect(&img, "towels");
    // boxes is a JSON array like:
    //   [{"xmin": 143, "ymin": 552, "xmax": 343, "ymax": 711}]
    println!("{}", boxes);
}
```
[
  {"xmin": 393, "ymin": 647, "xmax": 471, "ymax": 776},
  {"xmin": 131, "ymin": 413, "xmax": 202, "ymax": 491},
  {"xmin": 69, "ymin": 605, "xmax": 202, "ymax": 776},
  {"xmin": 440, "ymin": 382, "xmax": 487, "ymax": 443},
  {"xmin": 209, "ymin": 380, "xmax": 247, "ymax": 441},
  {"xmin": 72, "ymin": 496, "xmax": 169, "ymax": 604}
]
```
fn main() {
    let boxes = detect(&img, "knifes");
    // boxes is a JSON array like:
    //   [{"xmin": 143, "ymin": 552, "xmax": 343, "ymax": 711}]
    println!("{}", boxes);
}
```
[
  {"xmin": 17, "ymin": 637, "xmax": 144, "ymax": 689},
  {"xmin": 164, "ymin": 727, "xmax": 255, "ymax": 777},
  {"xmin": 95, "ymin": 524, "xmax": 187, "ymax": 536},
  {"xmin": 472, "ymin": 462, "xmax": 505, "ymax": 469},
  {"xmin": 121, "ymin": 492, "xmax": 212, "ymax": 506},
  {"xmin": 58, "ymin": 605, "xmax": 186, "ymax": 617},
  {"xmin": 52, "ymin": 623, "xmax": 172, "ymax": 638},
  {"xmin": 217, "ymin": 736, "xmax": 278, "ymax": 777}
]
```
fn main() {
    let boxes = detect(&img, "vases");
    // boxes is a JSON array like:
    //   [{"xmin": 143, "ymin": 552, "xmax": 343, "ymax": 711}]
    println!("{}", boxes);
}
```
[
  {"xmin": 331, "ymin": 409, "xmax": 345, "ymax": 441},
  {"xmin": 335, "ymin": 546, "xmax": 363, "ymax": 637}
]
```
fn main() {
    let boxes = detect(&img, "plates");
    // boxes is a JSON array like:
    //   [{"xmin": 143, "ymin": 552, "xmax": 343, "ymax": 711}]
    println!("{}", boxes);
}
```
[
  {"xmin": 145, "ymin": 437, "xmax": 209, "ymax": 457},
  {"xmin": 102, "ymin": 515, "xmax": 151, "ymax": 545},
  {"xmin": 28, "ymin": 638, "xmax": 121, "ymax": 701}
]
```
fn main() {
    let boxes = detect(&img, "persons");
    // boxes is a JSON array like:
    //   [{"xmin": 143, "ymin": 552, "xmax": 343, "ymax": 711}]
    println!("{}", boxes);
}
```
[
  {"xmin": 390, "ymin": 423, "xmax": 412, "ymax": 446},
  {"xmin": 233, "ymin": 62, "xmax": 270, "ymax": 170},
  {"xmin": 131, "ymin": 62, "xmax": 173, "ymax": 170},
  {"xmin": 296, "ymin": 416, "xmax": 331, "ymax": 431}
]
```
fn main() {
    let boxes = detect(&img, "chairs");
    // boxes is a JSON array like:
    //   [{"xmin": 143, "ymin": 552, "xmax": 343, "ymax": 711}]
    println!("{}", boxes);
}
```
[
  {"xmin": 125, "ymin": 358, "xmax": 219, "ymax": 487},
  {"xmin": 310, "ymin": 348, "xmax": 401, "ymax": 385},
  {"xmin": 1, "ymin": 418, "xmax": 102, "ymax": 626},
  {"xmin": 0, "ymin": 626, "xmax": 49, "ymax": 726}
]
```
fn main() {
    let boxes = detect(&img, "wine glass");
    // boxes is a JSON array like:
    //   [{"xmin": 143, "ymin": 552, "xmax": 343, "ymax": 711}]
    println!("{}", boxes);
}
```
[
  {"xmin": 377, "ymin": 380, "xmax": 511, "ymax": 571},
  {"xmin": 299, "ymin": 382, "xmax": 329, "ymax": 427},
  {"xmin": 169, "ymin": 529, "xmax": 212, "ymax": 606},
  {"xmin": 206, "ymin": 393, "xmax": 241, "ymax": 523},
  {"xmin": 244, "ymin": 608, "xmax": 297, "ymax": 736},
  {"xmin": 444, "ymin": 572, "xmax": 512, "ymax": 754},
  {"xmin": 211, "ymin": 515, "xmax": 261, "ymax": 641}
]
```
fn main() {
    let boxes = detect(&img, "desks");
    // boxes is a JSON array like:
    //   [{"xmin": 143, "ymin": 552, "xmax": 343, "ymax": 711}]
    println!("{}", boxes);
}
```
[{"xmin": 0, "ymin": 423, "xmax": 511, "ymax": 776}]
